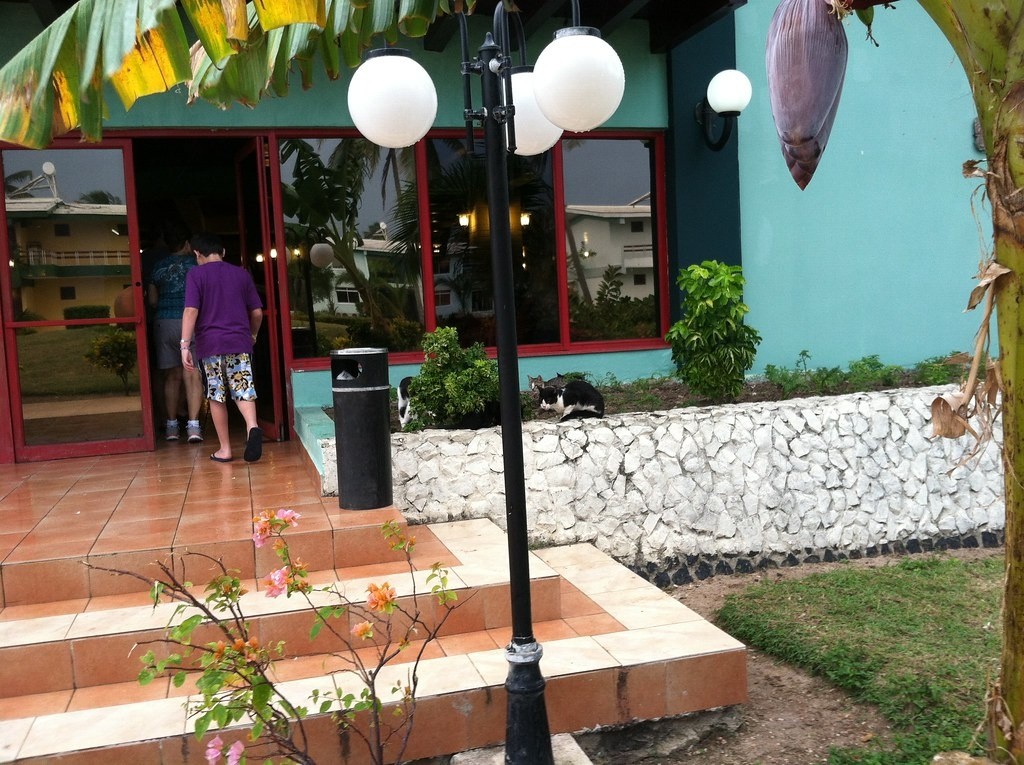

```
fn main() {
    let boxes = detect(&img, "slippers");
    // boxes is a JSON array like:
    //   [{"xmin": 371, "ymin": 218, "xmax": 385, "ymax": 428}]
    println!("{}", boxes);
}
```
[
  {"xmin": 211, "ymin": 451, "xmax": 232, "ymax": 463},
  {"xmin": 244, "ymin": 427, "xmax": 262, "ymax": 463}
]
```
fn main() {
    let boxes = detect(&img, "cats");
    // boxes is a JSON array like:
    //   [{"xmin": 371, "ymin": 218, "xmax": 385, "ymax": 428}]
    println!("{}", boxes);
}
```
[
  {"xmin": 397, "ymin": 376, "xmax": 427, "ymax": 428},
  {"xmin": 526, "ymin": 375, "xmax": 567, "ymax": 394},
  {"xmin": 535, "ymin": 380, "xmax": 605, "ymax": 423}
]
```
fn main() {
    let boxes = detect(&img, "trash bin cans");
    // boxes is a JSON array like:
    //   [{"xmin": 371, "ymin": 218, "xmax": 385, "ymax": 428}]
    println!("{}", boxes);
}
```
[{"xmin": 330, "ymin": 345, "xmax": 393, "ymax": 511}]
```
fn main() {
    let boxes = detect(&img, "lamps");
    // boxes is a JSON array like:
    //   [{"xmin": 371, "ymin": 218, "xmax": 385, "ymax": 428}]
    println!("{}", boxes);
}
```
[
  {"xmin": 520, "ymin": 212, "xmax": 533, "ymax": 228},
  {"xmin": 457, "ymin": 211, "xmax": 473, "ymax": 230},
  {"xmin": 693, "ymin": 69, "xmax": 752, "ymax": 153}
]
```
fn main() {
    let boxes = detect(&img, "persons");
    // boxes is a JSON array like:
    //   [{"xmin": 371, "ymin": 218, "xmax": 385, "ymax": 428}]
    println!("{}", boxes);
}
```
[
  {"xmin": 180, "ymin": 233, "xmax": 263, "ymax": 462},
  {"xmin": 144, "ymin": 229, "xmax": 203, "ymax": 443}
]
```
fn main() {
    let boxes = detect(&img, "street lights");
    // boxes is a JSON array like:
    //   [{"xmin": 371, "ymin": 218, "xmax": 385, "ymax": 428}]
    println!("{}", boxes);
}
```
[
  {"xmin": 276, "ymin": 223, "xmax": 335, "ymax": 357},
  {"xmin": 345, "ymin": 1, "xmax": 626, "ymax": 765}
]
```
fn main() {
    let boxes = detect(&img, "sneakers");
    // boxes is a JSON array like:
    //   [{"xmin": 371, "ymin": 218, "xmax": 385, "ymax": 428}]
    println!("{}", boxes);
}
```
[
  {"xmin": 187, "ymin": 426, "xmax": 204, "ymax": 443},
  {"xmin": 163, "ymin": 423, "xmax": 180, "ymax": 439}
]
